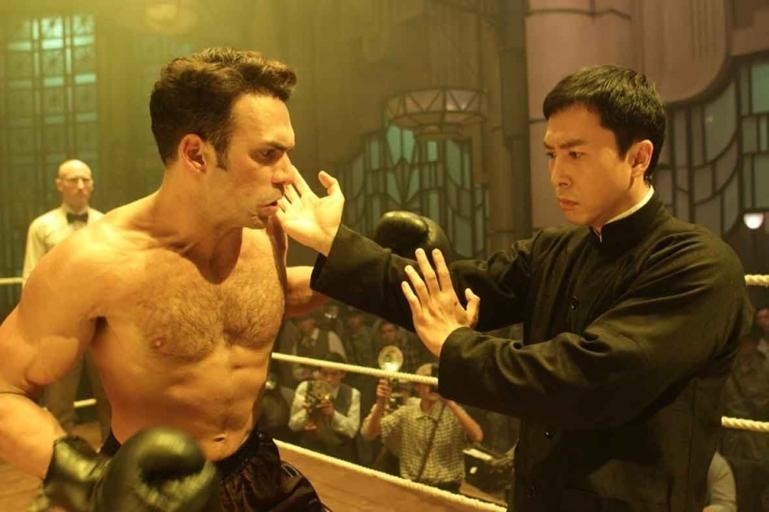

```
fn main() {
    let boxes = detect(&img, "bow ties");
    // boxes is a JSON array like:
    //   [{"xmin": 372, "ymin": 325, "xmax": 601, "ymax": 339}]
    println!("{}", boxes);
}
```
[{"xmin": 64, "ymin": 212, "xmax": 89, "ymax": 225}]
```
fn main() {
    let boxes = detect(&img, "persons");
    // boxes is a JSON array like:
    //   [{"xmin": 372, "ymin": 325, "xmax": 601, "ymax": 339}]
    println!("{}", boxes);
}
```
[
  {"xmin": 275, "ymin": 65, "xmax": 758, "ymax": 511},
  {"xmin": 259, "ymin": 303, "xmax": 423, "ymax": 473},
  {"xmin": 22, "ymin": 158, "xmax": 108, "ymax": 447},
  {"xmin": 0, "ymin": 47, "xmax": 455, "ymax": 512},
  {"xmin": 704, "ymin": 304, "xmax": 769, "ymax": 512},
  {"xmin": 361, "ymin": 363, "xmax": 484, "ymax": 494}
]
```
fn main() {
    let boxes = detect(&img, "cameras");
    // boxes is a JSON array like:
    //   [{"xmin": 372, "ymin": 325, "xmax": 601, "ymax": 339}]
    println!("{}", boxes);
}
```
[
  {"xmin": 305, "ymin": 382, "xmax": 334, "ymax": 415},
  {"xmin": 378, "ymin": 347, "xmax": 409, "ymax": 412}
]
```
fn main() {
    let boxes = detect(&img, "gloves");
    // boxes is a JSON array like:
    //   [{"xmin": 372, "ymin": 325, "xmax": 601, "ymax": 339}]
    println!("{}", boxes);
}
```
[
  {"xmin": 374, "ymin": 209, "xmax": 451, "ymax": 266},
  {"xmin": 39, "ymin": 422, "xmax": 222, "ymax": 512}
]
